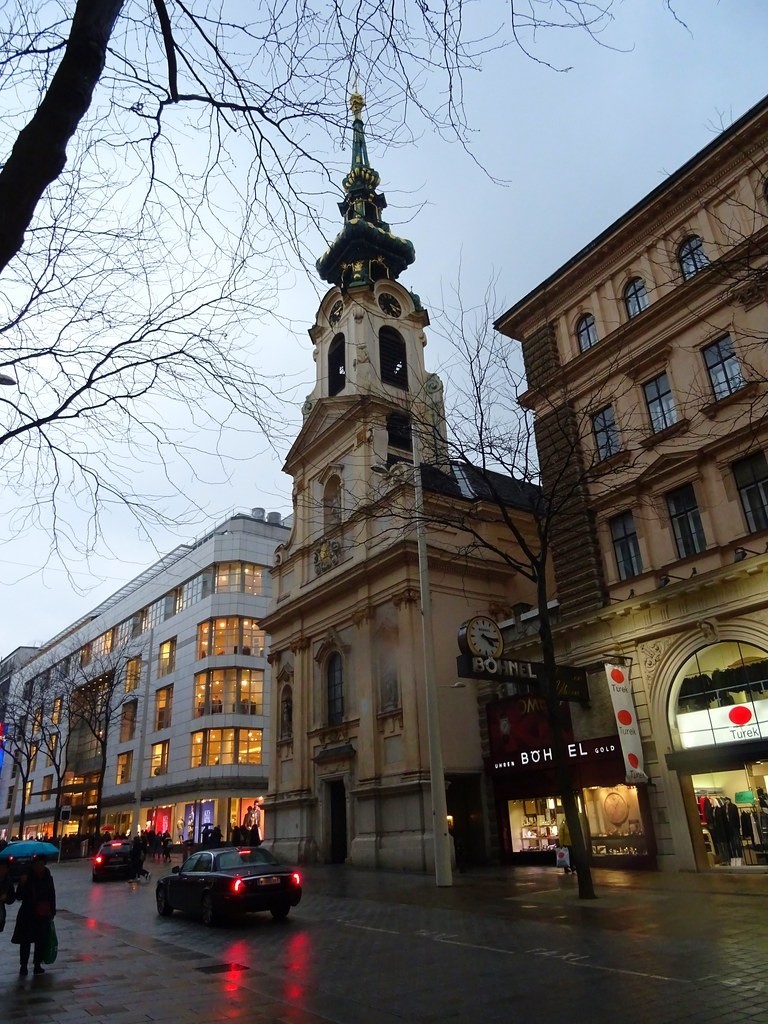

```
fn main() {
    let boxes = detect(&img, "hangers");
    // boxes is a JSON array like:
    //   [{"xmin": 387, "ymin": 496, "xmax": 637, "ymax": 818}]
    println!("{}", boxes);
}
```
[{"xmin": 740, "ymin": 807, "xmax": 757, "ymax": 813}]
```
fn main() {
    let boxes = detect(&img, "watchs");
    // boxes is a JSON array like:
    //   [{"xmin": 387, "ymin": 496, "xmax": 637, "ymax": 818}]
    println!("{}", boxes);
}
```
[{"xmin": 499, "ymin": 712, "xmax": 511, "ymax": 745}]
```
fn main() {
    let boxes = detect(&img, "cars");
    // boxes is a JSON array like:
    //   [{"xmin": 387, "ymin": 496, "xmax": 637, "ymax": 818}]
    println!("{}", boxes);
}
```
[
  {"xmin": 156, "ymin": 845, "xmax": 303, "ymax": 929},
  {"xmin": 90, "ymin": 839, "xmax": 137, "ymax": 882}
]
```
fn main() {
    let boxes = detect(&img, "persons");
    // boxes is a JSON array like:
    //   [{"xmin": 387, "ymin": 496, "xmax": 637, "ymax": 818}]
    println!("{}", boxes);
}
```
[
  {"xmin": 723, "ymin": 800, "xmax": 743, "ymax": 858},
  {"xmin": 243, "ymin": 800, "xmax": 262, "ymax": 841},
  {"xmin": 559, "ymin": 816, "xmax": 577, "ymax": 876},
  {"xmin": 0, "ymin": 823, "xmax": 261, "ymax": 976},
  {"xmin": 177, "ymin": 816, "xmax": 184, "ymax": 844}
]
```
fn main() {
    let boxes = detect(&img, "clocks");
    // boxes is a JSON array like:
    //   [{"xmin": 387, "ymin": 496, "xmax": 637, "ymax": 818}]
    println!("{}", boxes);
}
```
[
  {"xmin": 458, "ymin": 616, "xmax": 504, "ymax": 658},
  {"xmin": 604, "ymin": 792, "xmax": 629, "ymax": 823},
  {"xmin": 378, "ymin": 292, "xmax": 401, "ymax": 318},
  {"xmin": 329, "ymin": 300, "xmax": 343, "ymax": 326}
]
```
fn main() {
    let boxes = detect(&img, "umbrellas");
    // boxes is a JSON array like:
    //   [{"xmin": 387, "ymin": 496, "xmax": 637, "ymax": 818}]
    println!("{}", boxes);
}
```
[
  {"xmin": 101, "ymin": 826, "xmax": 114, "ymax": 830},
  {"xmin": 201, "ymin": 823, "xmax": 213, "ymax": 826},
  {"xmin": 0, "ymin": 841, "xmax": 59, "ymax": 859}
]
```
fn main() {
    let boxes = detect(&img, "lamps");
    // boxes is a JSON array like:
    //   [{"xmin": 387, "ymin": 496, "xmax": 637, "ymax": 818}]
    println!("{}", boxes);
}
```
[
  {"xmin": 530, "ymin": 798, "xmax": 534, "ymax": 804},
  {"xmin": 513, "ymin": 800, "xmax": 516, "ymax": 805}
]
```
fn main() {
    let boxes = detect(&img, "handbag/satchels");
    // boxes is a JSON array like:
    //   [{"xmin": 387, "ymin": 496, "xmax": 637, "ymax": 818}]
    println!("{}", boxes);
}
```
[
  {"xmin": 555, "ymin": 847, "xmax": 570, "ymax": 867},
  {"xmin": 44, "ymin": 918, "xmax": 58, "ymax": 964}
]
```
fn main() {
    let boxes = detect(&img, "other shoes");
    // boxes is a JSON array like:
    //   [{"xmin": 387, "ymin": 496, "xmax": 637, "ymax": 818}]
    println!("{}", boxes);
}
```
[
  {"xmin": 169, "ymin": 860, "xmax": 171, "ymax": 863},
  {"xmin": 146, "ymin": 872, "xmax": 151, "ymax": 881},
  {"xmin": 34, "ymin": 968, "xmax": 45, "ymax": 975},
  {"xmin": 136, "ymin": 877, "xmax": 141, "ymax": 881},
  {"xmin": 20, "ymin": 967, "xmax": 28, "ymax": 976},
  {"xmin": 565, "ymin": 866, "xmax": 576, "ymax": 872}
]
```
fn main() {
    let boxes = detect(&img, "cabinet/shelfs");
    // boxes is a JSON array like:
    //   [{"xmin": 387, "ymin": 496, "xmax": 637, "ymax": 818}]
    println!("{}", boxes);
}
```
[{"xmin": 520, "ymin": 798, "xmax": 559, "ymax": 851}]
